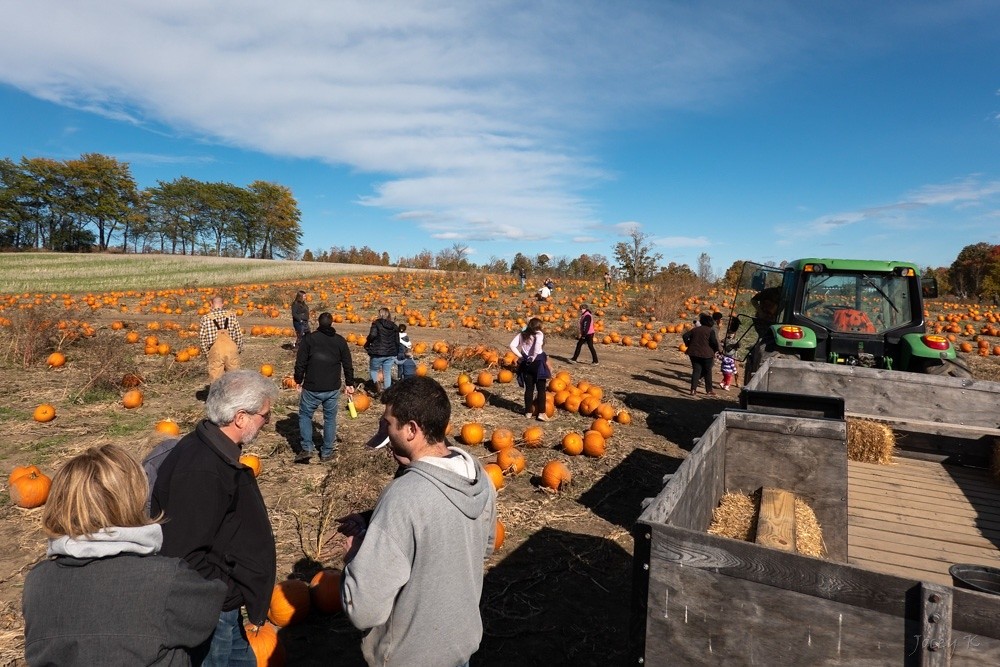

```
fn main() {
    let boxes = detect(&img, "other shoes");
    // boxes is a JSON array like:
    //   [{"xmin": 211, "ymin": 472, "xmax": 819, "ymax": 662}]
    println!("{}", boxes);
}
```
[
  {"xmin": 707, "ymin": 391, "xmax": 717, "ymax": 395},
  {"xmin": 691, "ymin": 389, "xmax": 697, "ymax": 395}
]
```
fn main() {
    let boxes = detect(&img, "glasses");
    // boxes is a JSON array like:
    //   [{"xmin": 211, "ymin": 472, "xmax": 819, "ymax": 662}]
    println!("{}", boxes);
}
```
[
  {"xmin": 245, "ymin": 410, "xmax": 272, "ymax": 420},
  {"xmin": 581, "ymin": 309, "xmax": 585, "ymax": 310}
]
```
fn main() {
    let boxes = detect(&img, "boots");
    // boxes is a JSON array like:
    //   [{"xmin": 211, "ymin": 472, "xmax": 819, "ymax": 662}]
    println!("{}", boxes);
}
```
[{"xmin": 374, "ymin": 380, "xmax": 383, "ymax": 398}]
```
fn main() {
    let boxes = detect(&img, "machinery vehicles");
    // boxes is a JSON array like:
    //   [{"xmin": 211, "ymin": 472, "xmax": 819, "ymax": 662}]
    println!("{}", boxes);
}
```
[{"xmin": 721, "ymin": 257, "xmax": 974, "ymax": 410}]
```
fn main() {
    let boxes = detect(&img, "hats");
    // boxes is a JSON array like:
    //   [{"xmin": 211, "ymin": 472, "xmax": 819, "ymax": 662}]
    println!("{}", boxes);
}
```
[
  {"xmin": 580, "ymin": 304, "xmax": 589, "ymax": 309},
  {"xmin": 699, "ymin": 313, "xmax": 714, "ymax": 327},
  {"xmin": 723, "ymin": 348, "xmax": 736, "ymax": 356},
  {"xmin": 713, "ymin": 312, "xmax": 723, "ymax": 320}
]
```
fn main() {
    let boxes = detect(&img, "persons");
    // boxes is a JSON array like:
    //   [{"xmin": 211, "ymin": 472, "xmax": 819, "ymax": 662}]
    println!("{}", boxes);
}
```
[
  {"xmin": 199, "ymin": 296, "xmax": 243, "ymax": 381},
  {"xmin": 519, "ymin": 267, "xmax": 526, "ymax": 289},
  {"xmin": 292, "ymin": 291, "xmax": 311, "ymax": 345},
  {"xmin": 24, "ymin": 444, "xmax": 226, "ymax": 667},
  {"xmin": 567, "ymin": 304, "xmax": 600, "ymax": 367},
  {"xmin": 536, "ymin": 278, "xmax": 554, "ymax": 300},
  {"xmin": 339, "ymin": 373, "xmax": 498, "ymax": 667},
  {"xmin": 509, "ymin": 315, "xmax": 551, "ymax": 421},
  {"xmin": 603, "ymin": 272, "xmax": 611, "ymax": 290},
  {"xmin": 363, "ymin": 308, "xmax": 417, "ymax": 392},
  {"xmin": 150, "ymin": 370, "xmax": 283, "ymax": 666},
  {"xmin": 681, "ymin": 311, "xmax": 742, "ymax": 397},
  {"xmin": 749, "ymin": 283, "xmax": 791, "ymax": 351},
  {"xmin": 293, "ymin": 313, "xmax": 354, "ymax": 463}
]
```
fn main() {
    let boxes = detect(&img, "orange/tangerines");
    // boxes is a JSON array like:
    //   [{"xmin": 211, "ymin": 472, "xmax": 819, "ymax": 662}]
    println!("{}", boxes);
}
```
[{"xmin": 0, "ymin": 272, "xmax": 1000, "ymax": 667}]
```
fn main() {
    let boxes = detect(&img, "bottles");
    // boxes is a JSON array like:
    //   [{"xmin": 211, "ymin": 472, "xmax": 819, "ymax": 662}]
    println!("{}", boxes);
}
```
[{"xmin": 348, "ymin": 395, "xmax": 358, "ymax": 418}]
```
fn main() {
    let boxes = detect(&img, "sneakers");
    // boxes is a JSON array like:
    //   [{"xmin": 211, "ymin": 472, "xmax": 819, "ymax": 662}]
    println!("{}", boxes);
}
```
[
  {"xmin": 568, "ymin": 359, "xmax": 577, "ymax": 363},
  {"xmin": 719, "ymin": 382, "xmax": 725, "ymax": 390},
  {"xmin": 538, "ymin": 414, "xmax": 550, "ymax": 421},
  {"xmin": 589, "ymin": 362, "xmax": 599, "ymax": 366},
  {"xmin": 724, "ymin": 386, "xmax": 731, "ymax": 391},
  {"xmin": 321, "ymin": 451, "xmax": 337, "ymax": 461},
  {"xmin": 525, "ymin": 412, "xmax": 533, "ymax": 418},
  {"xmin": 294, "ymin": 450, "xmax": 312, "ymax": 462}
]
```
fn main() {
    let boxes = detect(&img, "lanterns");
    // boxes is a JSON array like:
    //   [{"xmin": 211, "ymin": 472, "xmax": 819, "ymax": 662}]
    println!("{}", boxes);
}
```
[{"xmin": 833, "ymin": 310, "xmax": 876, "ymax": 335}]
